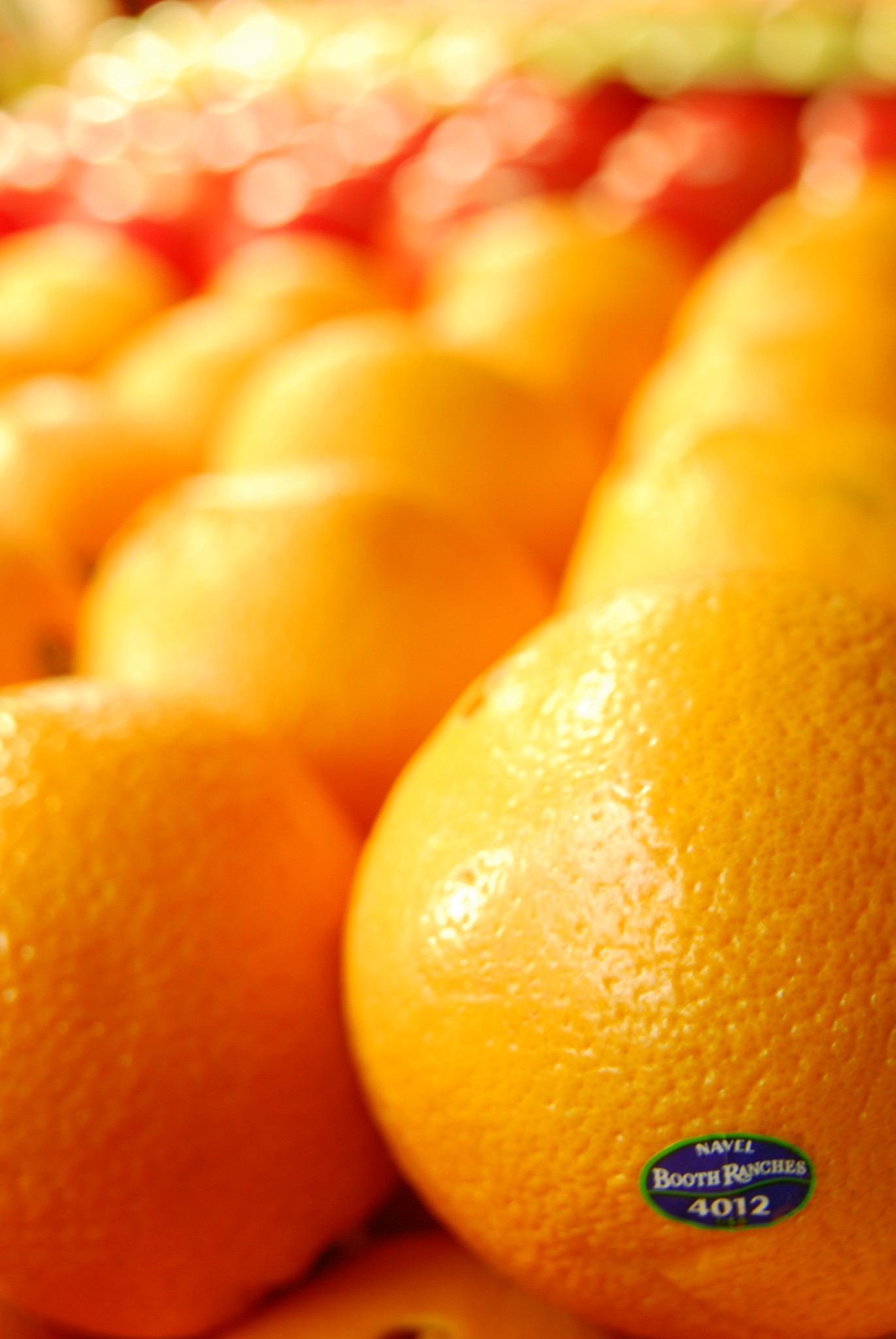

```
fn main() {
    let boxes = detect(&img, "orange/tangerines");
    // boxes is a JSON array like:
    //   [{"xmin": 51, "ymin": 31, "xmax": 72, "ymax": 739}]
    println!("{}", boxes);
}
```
[{"xmin": 0, "ymin": 170, "xmax": 896, "ymax": 1339}]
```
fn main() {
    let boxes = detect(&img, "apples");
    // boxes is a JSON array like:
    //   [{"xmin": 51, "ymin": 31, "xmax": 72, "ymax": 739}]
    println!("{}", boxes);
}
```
[{"xmin": 0, "ymin": 63, "xmax": 896, "ymax": 295}]
